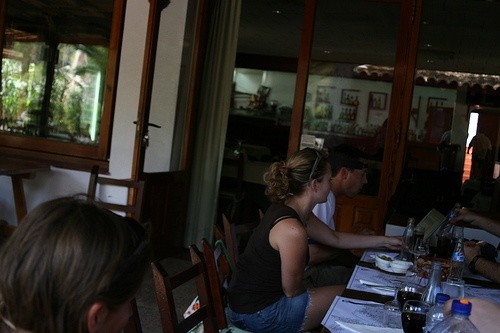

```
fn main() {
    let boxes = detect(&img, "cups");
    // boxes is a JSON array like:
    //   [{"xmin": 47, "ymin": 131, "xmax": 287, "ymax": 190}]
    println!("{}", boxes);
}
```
[
  {"xmin": 401, "ymin": 300, "xmax": 430, "ymax": 333},
  {"xmin": 443, "ymin": 277, "xmax": 464, "ymax": 300},
  {"xmin": 398, "ymin": 272, "xmax": 427, "ymax": 305}
]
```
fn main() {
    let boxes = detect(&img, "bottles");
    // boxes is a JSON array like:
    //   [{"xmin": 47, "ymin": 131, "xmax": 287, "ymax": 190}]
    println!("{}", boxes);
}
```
[
  {"xmin": 408, "ymin": 128, "xmax": 415, "ymax": 142},
  {"xmin": 425, "ymin": 293, "xmax": 451, "ymax": 333},
  {"xmin": 423, "ymin": 260, "xmax": 442, "ymax": 324},
  {"xmin": 333, "ymin": 120, "xmax": 342, "ymax": 133},
  {"xmin": 446, "ymin": 239, "xmax": 466, "ymax": 285},
  {"xmin": 345, "ymin": 94, "xmax": 359, "ymax": 105},
  {"xmin": 340, "ymin": 108, "xmax": 355, "ymax": 123},
  {"xmin": 401, "ymin": 218, "xmax": 415, "ymax": 258},
  {"xmin": 418, "ymin": 129, "xmax": 424, "ymax": 140},
  {"xmin": 430, "ymin": 300, "xmax": 480, "ymax": 333},
  {"xmin": 373, "ymin": 97, "xmax": 381, "ymax": 108}
]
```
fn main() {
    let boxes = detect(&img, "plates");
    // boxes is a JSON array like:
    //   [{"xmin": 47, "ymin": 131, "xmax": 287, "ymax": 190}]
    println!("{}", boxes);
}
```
[{"xmin": 377, "ymin": 261, "xmax": 414, "ymax": 275}]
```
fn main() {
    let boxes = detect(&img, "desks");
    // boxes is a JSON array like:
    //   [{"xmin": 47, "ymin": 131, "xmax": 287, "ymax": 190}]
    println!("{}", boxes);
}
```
[{"xmin": 320, "ymin": 232, "xmax": 500, "ymax": 333}]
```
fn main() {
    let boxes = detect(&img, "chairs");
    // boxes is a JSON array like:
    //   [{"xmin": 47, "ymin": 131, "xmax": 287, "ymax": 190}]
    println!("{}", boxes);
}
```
[
  {"xmin": 86, "ymin": 164, "xmax": 147, "ymax": 223},
  {"xmin": 151, "ymin": 209, "xmax": 265, "ymax": 333}
]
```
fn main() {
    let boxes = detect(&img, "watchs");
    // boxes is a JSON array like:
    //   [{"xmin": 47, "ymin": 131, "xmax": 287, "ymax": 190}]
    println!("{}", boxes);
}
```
[{"xmin": 468, "ymin": 255, "xmax": 481, "ymax": 273}]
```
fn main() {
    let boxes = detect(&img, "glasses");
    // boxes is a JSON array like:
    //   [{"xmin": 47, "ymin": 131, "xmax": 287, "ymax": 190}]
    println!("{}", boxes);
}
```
[{"xmin": 72, "ymin": 192, "xmax": 150, "ymax": 297}]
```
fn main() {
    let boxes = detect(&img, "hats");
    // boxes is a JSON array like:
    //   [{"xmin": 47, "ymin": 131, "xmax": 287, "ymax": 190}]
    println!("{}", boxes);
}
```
[{"xmin": 309, "ymin": 148, "xmax": 328, "ymax": 182}]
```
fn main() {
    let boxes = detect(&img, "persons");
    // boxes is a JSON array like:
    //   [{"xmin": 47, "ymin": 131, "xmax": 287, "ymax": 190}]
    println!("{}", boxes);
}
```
[
  {"xmin": 226, "ymin": 119, "xmax": 500, "ymax": 333},
  {"xmin": 0, "ymin": 197, "xmax": 149, "ymax": 333}
]
